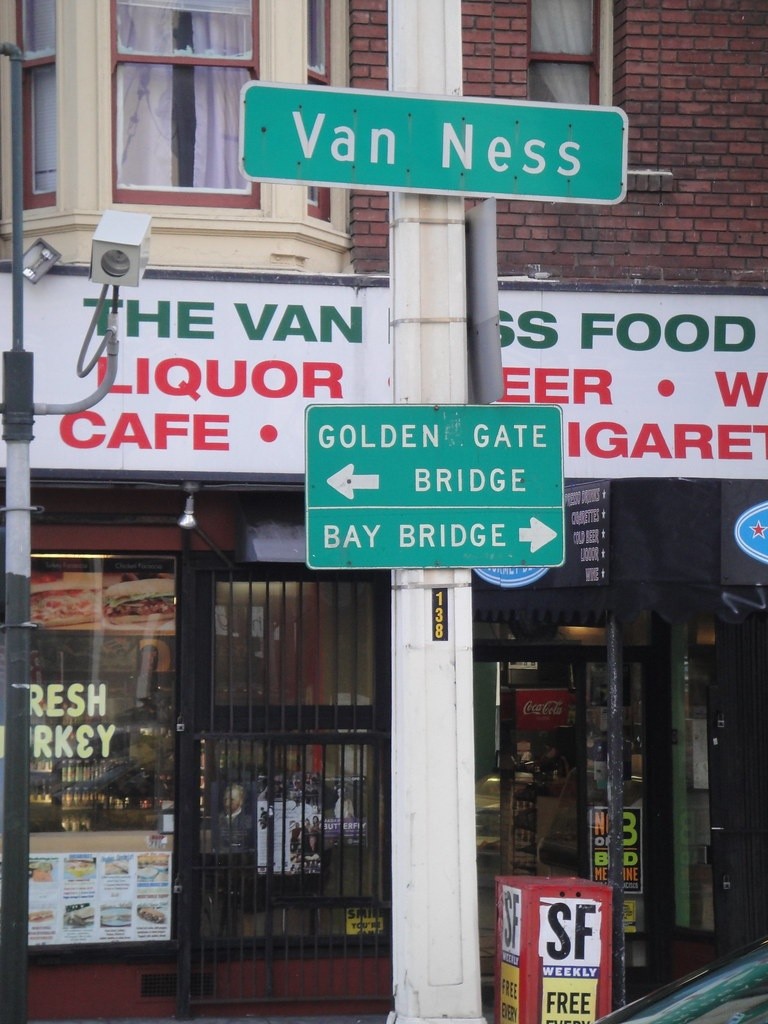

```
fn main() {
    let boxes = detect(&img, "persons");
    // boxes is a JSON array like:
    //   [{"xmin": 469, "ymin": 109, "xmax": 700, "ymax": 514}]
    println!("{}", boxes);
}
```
[
  {"xmin": 259, "ymin": 807, "xmax": 267, "ymax": 829},
  {"xmin": 207, "ymin": 784, "xmax": 251, "ymax": 853},
  {"xmin": 291, "ymin": 816, "xmax": 320, "ymax": 875},
  {"xmin": 334, "ymin": 788, "xmax": 354, "ymax": 845}
]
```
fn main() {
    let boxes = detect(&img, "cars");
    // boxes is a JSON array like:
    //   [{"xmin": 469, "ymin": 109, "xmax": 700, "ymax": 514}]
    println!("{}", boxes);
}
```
[{"xmin": 476, "ymin": 773, "xmax": 534, "ymax": 888}]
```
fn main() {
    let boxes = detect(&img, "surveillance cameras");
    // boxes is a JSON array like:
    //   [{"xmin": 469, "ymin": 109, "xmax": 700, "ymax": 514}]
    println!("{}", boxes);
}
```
[{"xmin": 88, "ymin": 209, "xmax": 151, "ymax": 287}]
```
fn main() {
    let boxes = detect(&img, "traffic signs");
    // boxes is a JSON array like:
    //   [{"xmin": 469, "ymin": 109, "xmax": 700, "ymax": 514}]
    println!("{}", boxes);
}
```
[{"xmin": 306, "ymin": 404, "xmax": 568, "ymax": 570}]
